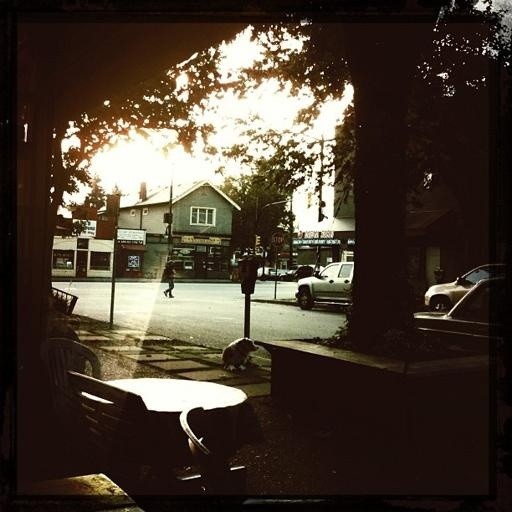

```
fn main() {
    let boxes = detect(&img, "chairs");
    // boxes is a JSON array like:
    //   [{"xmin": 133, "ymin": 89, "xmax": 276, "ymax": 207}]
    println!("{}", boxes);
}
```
[{"xmin": 41, "ymin": 323, "xmax": 219, "ymax": 494}]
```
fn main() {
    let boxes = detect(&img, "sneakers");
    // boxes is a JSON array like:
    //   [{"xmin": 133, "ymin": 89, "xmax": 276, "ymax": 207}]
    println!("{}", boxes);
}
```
[{"xmin": 164, "ymin": 291, "xmax": 174, "ymax": 298}]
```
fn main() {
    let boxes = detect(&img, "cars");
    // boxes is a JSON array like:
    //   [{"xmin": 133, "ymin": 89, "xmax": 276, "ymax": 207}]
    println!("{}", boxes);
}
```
[
  {"xmin": 294, "ymin": 261, "xmax": 355, "ymax": 310},
  {"xmin": 409, "ymin": 277, "xmax": 511, "ymax": 361},
  {"xmin": 424, "ymin": 262, "xmax": 511, "ymax": 313},
  {"xmin": 257, "ymin": 264, "xmax": 324, "ymax": 282}
]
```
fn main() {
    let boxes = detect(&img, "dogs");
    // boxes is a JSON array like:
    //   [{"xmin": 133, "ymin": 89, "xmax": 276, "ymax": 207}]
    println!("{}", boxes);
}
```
[{"xmin": 222, "ymin": 337, "xmax": 260, "ymax": 371}]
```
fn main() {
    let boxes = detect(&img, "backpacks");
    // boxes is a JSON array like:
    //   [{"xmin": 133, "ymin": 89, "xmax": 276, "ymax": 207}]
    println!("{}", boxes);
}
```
[{"xmin": 162, "ymin": 269, "xmax": 168, "ymax": 282}]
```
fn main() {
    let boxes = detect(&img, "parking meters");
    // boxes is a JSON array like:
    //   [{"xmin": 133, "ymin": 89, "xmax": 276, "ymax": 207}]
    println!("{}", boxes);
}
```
[{"xmin": 237, "ymin": 254, "xmax": 265, "ymax": 339}]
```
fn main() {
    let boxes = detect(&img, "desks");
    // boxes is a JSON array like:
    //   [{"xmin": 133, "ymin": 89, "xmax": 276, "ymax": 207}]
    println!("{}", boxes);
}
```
[{"xmin": 80, "ymin": 377, "xmax": 263, "ymax": 495}]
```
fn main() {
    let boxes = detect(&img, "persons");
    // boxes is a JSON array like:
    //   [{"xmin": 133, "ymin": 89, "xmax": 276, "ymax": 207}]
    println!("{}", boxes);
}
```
[{"xmin": 163, "ymin": 263, "xmax": 175, "ymax": 298}]
